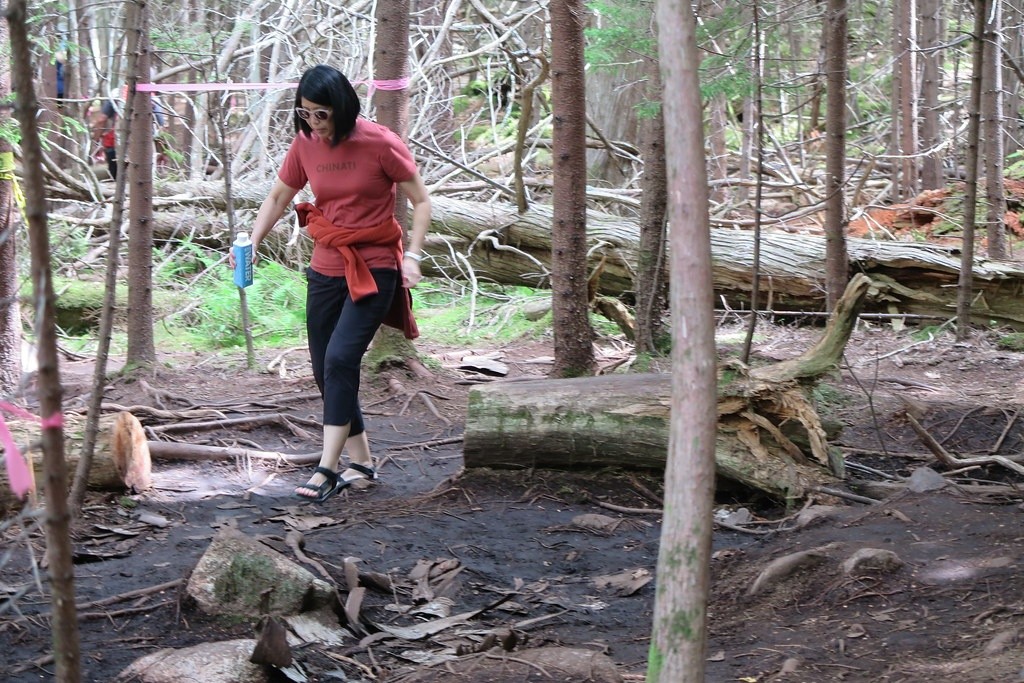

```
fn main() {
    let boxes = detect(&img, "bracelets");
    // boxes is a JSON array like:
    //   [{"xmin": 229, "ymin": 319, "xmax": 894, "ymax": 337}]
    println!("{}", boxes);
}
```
[{"xmin": 404, "ymin": 251, "xmax": 421, "ymax": 261}]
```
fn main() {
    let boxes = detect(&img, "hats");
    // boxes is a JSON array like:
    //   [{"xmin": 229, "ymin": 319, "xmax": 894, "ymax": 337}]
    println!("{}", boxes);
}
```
[{"xmin": 110, "ymin": 88, "xmax": 120, "ymax": 98}]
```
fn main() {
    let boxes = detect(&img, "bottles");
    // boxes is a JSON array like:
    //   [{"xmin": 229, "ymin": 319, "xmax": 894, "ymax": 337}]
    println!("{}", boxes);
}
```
[{"xmin": 232, "ymin": 232, "xmax": 253, "ymax": 289}]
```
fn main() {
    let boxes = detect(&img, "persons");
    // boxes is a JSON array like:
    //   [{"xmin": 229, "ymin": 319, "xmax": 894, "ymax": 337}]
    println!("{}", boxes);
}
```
[
  {"xmin": 92, "ymin": 67, "xmax": 165, "ymax": 180},
  {"xmin": 229, "ymin": 66, "xmax": 431, "ymax": 503},
  {"xmin": 56, "ymin": 27, "xmax": 71, "ymax": 108}
]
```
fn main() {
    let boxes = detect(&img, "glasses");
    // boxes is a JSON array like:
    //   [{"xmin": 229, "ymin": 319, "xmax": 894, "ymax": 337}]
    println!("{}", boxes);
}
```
[{"xmin": 295, "ymin": 106, "xmax": 332, "ymax": 120}]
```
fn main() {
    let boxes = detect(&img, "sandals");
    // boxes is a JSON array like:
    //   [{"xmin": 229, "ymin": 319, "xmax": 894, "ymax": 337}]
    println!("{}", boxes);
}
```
[
  {"xmin": 296, "ymin": 467, "xmax": 338, "ymax": 503},
  {"xmin": 337, "ymin": 462, "xmax": 379, "ymax": 492}
]
